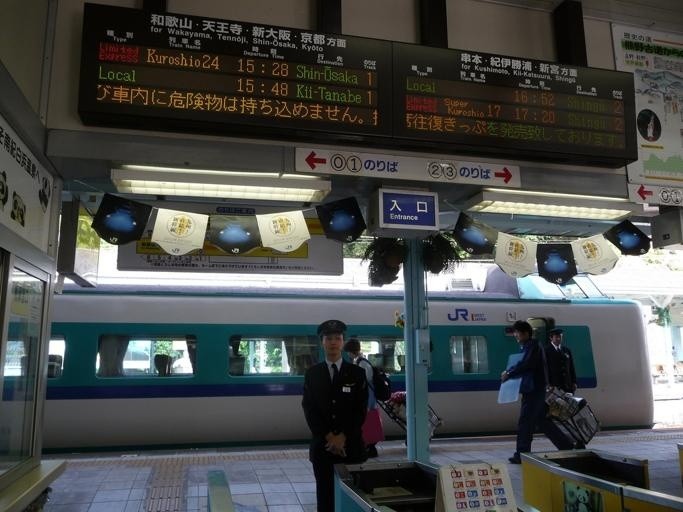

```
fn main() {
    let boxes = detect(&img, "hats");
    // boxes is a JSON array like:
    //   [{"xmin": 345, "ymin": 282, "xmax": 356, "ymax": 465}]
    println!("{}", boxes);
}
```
[
  {"xmin": 318, "ymin": 320, "xmax": 346, "ymax": 334},
  {"xmin": 548, "ymin": 329, "xmax": 563, "ymax": 335}
]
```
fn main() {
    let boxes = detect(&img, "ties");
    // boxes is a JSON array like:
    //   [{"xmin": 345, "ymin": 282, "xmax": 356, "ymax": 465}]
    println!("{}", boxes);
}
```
[
  {"xmin": 332, "ymin": 363, "xmax": 338, "ymax": 382},
  {"xmin": 557, "ymin": 346, "xmax": 560, "ymax": 352}
]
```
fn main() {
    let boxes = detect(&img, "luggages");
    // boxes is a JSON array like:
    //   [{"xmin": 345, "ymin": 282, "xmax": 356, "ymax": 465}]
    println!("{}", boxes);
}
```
[
  {"xmin": 545, "ymin": 385, "xmax": 600, "ymax": 445},
  {"xmin": 387, "ymin": 392, "xmax": 442, "ymax": 438}
]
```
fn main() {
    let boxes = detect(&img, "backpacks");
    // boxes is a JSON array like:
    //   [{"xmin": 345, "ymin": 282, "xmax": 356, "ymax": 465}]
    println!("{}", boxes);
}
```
[{"xmin": 357, "ymin": 358, "xmax": 391, "ymax": 401}]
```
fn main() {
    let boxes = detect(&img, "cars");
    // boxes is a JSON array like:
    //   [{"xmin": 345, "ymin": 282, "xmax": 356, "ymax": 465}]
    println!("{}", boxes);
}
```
[{"xmin": 123, "ymin": 350, "xmax": 149, "ymax": 374}]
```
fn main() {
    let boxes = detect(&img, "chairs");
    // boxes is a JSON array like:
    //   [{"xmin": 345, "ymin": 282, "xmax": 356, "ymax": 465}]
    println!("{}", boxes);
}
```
[
  {"xmin": 153, "ymin": 354, "xmax": 172, "ymax": 377},
  {"xmin": 287, "ymin": 353, "xmax": 310, "ymax": 377}
]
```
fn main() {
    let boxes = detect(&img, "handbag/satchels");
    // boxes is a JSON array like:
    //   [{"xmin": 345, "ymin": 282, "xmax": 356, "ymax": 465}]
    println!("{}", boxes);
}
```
[{"xmin": 363, "ymin": 409, "xmax": 384, "ymax": 444}]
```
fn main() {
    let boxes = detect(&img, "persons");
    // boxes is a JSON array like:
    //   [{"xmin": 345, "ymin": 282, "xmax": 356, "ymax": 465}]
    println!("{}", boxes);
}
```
[
  {"xmin": 301, "ymin": 319, "xmax": 369, "ymax": 512},
  {"xmin": 501, "ymin": 321, "xmax": 574, "ymax": 463},
  {"xmin": 546, "ymin": 328, "xmax": 578, "ymax": 393},
  {"xmin": 342, "ymin": 337, "xmax": 379, "ymax": 457}
]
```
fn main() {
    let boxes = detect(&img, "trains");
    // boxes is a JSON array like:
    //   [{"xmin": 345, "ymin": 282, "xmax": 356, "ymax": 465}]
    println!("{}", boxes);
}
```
[
  {"xmin": 0, "ymin": 281, "xmax": 41, "ymax": 460},
  {"xmin": 43, "ymin": 273, "xmax": 655, "ymax": 455}
]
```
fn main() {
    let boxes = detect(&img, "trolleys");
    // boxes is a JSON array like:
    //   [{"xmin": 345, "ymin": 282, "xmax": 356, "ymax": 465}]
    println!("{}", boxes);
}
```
[
  {"xmin": 547, "ymin": 414, "xmax": 599, "ymax": 449},
  {"xmin": 377, "ymin": 399, "xmax": 443, "ymax": 448}
]
